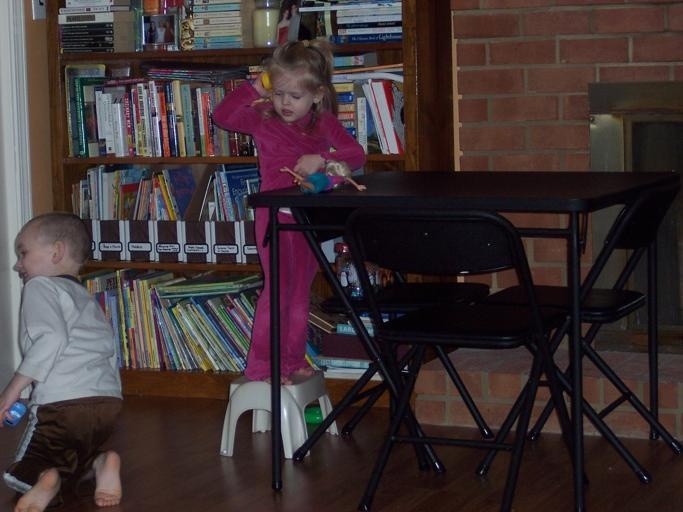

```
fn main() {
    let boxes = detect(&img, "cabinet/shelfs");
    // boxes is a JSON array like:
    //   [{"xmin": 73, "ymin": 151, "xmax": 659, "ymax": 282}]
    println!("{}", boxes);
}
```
[{"xmin": 23, "ymin": 2, "xmax": 452, "ymax": 408}]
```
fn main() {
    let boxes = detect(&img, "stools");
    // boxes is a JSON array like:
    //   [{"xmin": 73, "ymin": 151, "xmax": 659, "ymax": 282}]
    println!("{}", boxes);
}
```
[{"xmin": 219, "ymin": 371, "xmax": 339, "ymax": 460}]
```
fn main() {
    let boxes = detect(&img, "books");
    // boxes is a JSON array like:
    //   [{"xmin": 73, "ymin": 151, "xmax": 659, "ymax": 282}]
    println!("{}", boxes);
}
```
[
  {"xmin": 76, "ymin": 268, "xmax": 406, "ymax": 379},
  {"xmin": 56, "ymin": 1, "xmax": 404, "ymax": 54},
  {"xmin": 63, "ymin": 55, "xmax": 406, "ymax": 158},
  {"xmin": 69, "ymin": 163, "xmax": 261, "ymax": 221}
]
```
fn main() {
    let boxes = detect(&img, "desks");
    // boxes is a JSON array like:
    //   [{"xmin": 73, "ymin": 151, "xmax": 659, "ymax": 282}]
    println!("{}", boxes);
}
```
[{"xmin": 249, "ymin": 169, "xmax": 683, "ymax": 512}]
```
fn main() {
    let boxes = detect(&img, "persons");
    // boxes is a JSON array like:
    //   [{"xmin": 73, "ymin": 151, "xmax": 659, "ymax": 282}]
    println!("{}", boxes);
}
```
[
  {"xmin": 208, "ymin": 38, "xmax": 366, "ymax": 388},
  {"xmin": 0, "ymin": 212, "xmax": 125, "ymax": 511}
]
